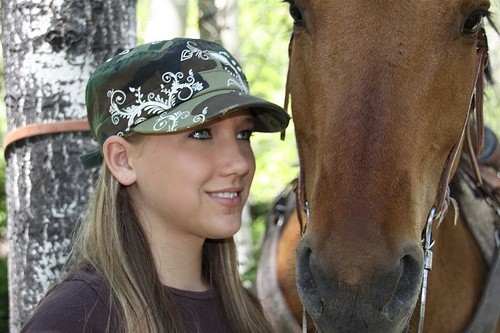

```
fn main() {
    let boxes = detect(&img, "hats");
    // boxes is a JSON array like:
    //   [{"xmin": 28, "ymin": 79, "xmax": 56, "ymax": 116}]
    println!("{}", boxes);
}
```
[{"xmin": 80, "ymin": 37, "xmax": 289, "ymax": 170}]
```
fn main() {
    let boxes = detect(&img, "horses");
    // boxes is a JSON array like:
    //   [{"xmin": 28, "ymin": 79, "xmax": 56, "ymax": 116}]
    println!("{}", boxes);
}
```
[{"xmin": 252, "ymin": 0, "xmax": 500, "ymax": 333}]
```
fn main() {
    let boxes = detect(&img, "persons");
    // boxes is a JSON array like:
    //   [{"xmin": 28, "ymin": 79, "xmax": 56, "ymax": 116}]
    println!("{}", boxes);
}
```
[{"xmin": 19, "ymin": 37, "xmax": 290, "ymax": 333}]
